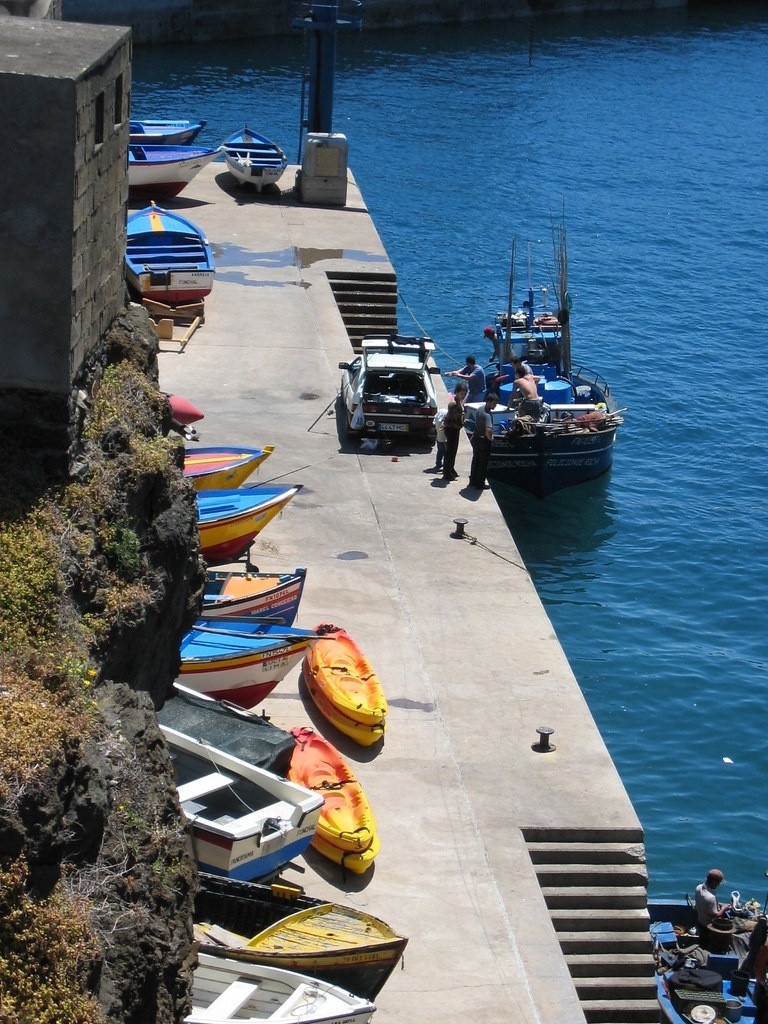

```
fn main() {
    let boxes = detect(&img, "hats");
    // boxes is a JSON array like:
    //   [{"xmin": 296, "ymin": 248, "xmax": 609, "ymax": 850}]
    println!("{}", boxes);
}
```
[
  {"xmin": 708, "ymin": 869, "xmax": 727, "ymax": 883},
  {"xmin": 484, "ymin": 326, "xmax": 493, "ymax": 337}
]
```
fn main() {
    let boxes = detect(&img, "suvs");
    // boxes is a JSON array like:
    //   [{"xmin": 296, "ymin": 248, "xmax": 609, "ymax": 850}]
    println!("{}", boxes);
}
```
[{"xmin": 338, "ymin": 332, "xmax": 442, "ymax": 448}]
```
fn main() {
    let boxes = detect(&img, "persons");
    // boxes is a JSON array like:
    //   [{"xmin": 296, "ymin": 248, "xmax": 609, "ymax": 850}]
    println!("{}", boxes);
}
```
[
  {"xmin": 483, "ymin": 326, "xmax": 517, "ymax": 364},
  {"xmin": 468, "ymin": 393, "xmax": 500, "ymax": 490},
  {"xmin": 433, "ymin": 409, "xmax": 448, "ymax": 472},
  {"xmin": 695, "ymin": 868, "xmax": 733, "ymax": 945},
  {"xmin": 508, "ymin": 356, "xmax": 534, "ymax": 408},
  {"xmin": 504, "ymin": 366, "xmax": 540, "ymax": 419},
  {"xmin": 439, "ymin": 383, "xmax": 468, "ymax": 482},
  {"xmin": 444, "ymin": 355, "xmax": 485, "ymax": 403}
]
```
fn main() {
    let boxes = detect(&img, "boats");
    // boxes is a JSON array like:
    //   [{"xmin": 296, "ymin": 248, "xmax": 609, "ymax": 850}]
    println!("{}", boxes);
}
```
[
  {"xmin": 220, "ymin": 121, "xmax": 289, "ymax": 194},
  {"xmin": 646, "ymin": 896, "xmax": 768, "ymax": 1024},
  {"xmin": 154, "ymin": 681, "xmax": 299, "ymax": 779},
  {"xmin": 301, "ymin": 620, "xmax": 389, "ymax": 749},
  {"xmin": 193, "ymin": 482, "xmax": 305, "ymax": 565},
  {"xmin": 128, "ymin": 144, "xmax": 227, "ymax": 201},
  {"xmin": 196, "ymin": 564, "xmax": 307, "ymax": 628},
  {"xmin": 186, "ymin": 867, "xmax": 410, "ymax": 1004},
  {"xmin": 280, "ymin": 725, "xmax": 381, "ymax": 876},
  {"xmin": 175, "ymin": 950, "xmax": 377, "ymax": 1024},
  {"xmin": 171, "ymin": 614, "xmax": 320, "ymax": 711},
  {"xmin": 128, "ymin": 118, "xmax": 208, "ymax": 147},
  {"xmin": 181, "ymin": 444, "xmax": 275, "ymax": 491},
  {"xmin": 158, "ymin": 723, "xmax": 326, "ymax": 887},
  {"xmin": 122, "ymin": 199, "xmax": 216, "ymax": 304},
  {"xmin": 454, "ymin": 193, "xmax": 629, "ymax": 502}
]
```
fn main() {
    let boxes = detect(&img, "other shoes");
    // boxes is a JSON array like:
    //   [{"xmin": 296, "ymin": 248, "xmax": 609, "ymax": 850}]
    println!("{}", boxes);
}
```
[
  {"xmin": 483, "ymin": 485, "xmax": 491, "ymax": 489},
  {"xmin": 437, "ymin": 467, "xmax": 443, "ymax": 472}
]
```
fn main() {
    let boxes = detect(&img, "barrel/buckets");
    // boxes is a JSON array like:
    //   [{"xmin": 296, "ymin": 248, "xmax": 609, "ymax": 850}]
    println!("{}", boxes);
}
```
[
  {"xmin": 725, "ymin": 1000, "xmax": 742, "ymax": 1022},
  {"xmin": 711, "ymin": 918, "xmax": 733, "ymax": 930},
  {"xmin": 730, "ymin": 970, "xmax": 750, "ymax": 995}
]
